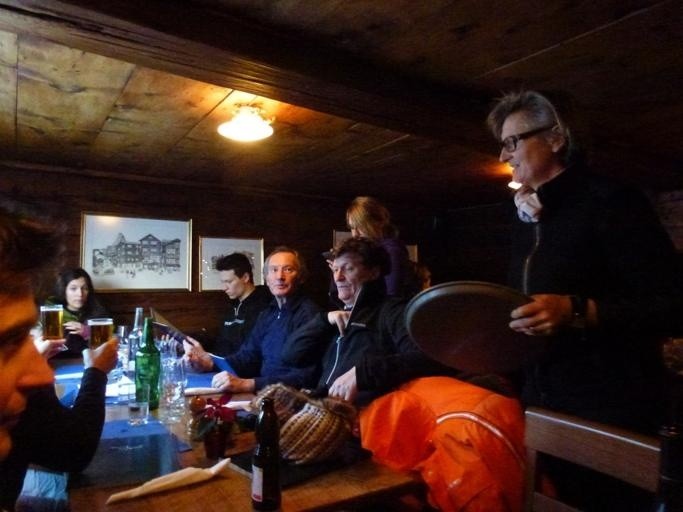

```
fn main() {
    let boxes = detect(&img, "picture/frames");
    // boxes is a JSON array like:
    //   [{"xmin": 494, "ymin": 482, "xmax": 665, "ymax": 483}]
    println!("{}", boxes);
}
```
[
  {"xmin": 198, "ymin": 235, "xmax": 264, "ymax": 293},
  {"xmin": 333, "ymin": 229, "xmax": 354, "ymax": 249},
  {"xmin": 78, "ymin": 212, "xmax": 193, "ymax": 296}
]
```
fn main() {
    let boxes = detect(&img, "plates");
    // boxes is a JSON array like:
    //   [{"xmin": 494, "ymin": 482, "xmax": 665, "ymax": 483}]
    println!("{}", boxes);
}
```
[{"xmin": 400, "ymin": 280, "xmax": 550, "ymax": 377}]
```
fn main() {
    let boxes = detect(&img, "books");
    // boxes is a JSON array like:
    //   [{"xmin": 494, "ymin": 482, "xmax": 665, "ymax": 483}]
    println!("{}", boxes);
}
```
[
  {"xmin": 206, "ymin": 350, "xmax": 240, "ymax": 379},
  {"xmin": 147, "ymin": 305, "xmax": 193, "ymax": 348}
]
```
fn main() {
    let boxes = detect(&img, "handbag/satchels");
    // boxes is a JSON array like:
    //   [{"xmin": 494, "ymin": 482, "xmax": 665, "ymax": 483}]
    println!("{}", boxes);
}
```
[{"xmin": 248, "ymin": 381, "xmax": 359, "ymax": 469}]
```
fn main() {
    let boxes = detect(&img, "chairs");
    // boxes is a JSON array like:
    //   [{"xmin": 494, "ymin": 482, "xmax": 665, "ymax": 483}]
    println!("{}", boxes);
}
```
[{"xmin": 418, "ymin": 375, "xmax": 667, "ymax": 512}]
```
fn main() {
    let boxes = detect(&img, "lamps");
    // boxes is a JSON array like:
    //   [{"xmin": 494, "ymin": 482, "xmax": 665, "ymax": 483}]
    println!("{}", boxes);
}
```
[{"xmin": 217, "ymin": 105, "xmax": 274, "ymax": 142}]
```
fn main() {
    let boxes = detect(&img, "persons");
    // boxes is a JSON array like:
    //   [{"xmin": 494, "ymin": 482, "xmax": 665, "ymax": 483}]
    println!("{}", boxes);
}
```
[
  {"xmin": 183, "ymin": 243, "xmax": 325, "ymax": 398},
  {"xmin": 344, "ymin": 192, "xmax": 415, "ymax": 302},
  {"xmin": 0, "ymin": 336, "xmax": 123, "ymax": 512},
  {"xmin": 232, "ymin": 235, "xmax": 453, "ymax": 415},
  {"xmin": 480, "ymin": 86, "xmax": 682, "ymax": 510},
  {"xmin": 158, "ymin": 252, "xmax": 275, "ymax": 357},
  {"xmin": 1, "ymin": 201, "xmax": 70, "ymax": 463},
  {"xmin": 40, "ymin": 263, "xmax": 115, "ymax": 356}
]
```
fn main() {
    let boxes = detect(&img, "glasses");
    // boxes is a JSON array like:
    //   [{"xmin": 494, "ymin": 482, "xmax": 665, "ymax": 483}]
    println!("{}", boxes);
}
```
[{"xmin": 495, "ymin": 125, "xmax": 555, "ymax": 152}]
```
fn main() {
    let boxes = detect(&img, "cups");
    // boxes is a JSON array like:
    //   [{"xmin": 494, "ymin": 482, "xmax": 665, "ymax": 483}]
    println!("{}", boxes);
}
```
[
  {"xmin": 38, "ymin": 303, "xmax": 63, "ymax": 341},
  {"xmin": 127, "ymin": 385, "xmax": 150, "ymax": 424},
  {"xmin": 160, "ymin": 340, "xmax": 176, "ymax": 366},
  {"xmin": 86, "ymin": 317, "xmax": 113, "ymax": 350}
]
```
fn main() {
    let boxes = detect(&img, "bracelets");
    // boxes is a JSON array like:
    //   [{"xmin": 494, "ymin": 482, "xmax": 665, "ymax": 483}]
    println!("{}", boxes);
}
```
[{"xmin": 570, "ymin": 290, "xmax": 589, "ymax": 321}]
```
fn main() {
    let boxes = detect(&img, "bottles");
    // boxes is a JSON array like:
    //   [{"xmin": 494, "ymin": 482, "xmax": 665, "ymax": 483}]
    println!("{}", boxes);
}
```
[
  {"xmin": 251, "ymin": 398, "xmax": 283, "ymax": 509},
  {"xmin": 116, "ymin": 326, "xmax": 130, "ymax": 368},
  {"xmin": 127, "ymin": 307, "xmax": 145, "ymax": 361},
  {"xmin": 134, "ymin": 316, "xmax": 160, "ymax": 411}
]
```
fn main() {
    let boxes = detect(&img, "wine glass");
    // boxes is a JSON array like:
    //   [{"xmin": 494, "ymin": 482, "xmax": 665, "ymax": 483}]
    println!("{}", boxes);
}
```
[{"xmin": 158, "ymin": 355, "xmax": 186, "ymax": 425}]
